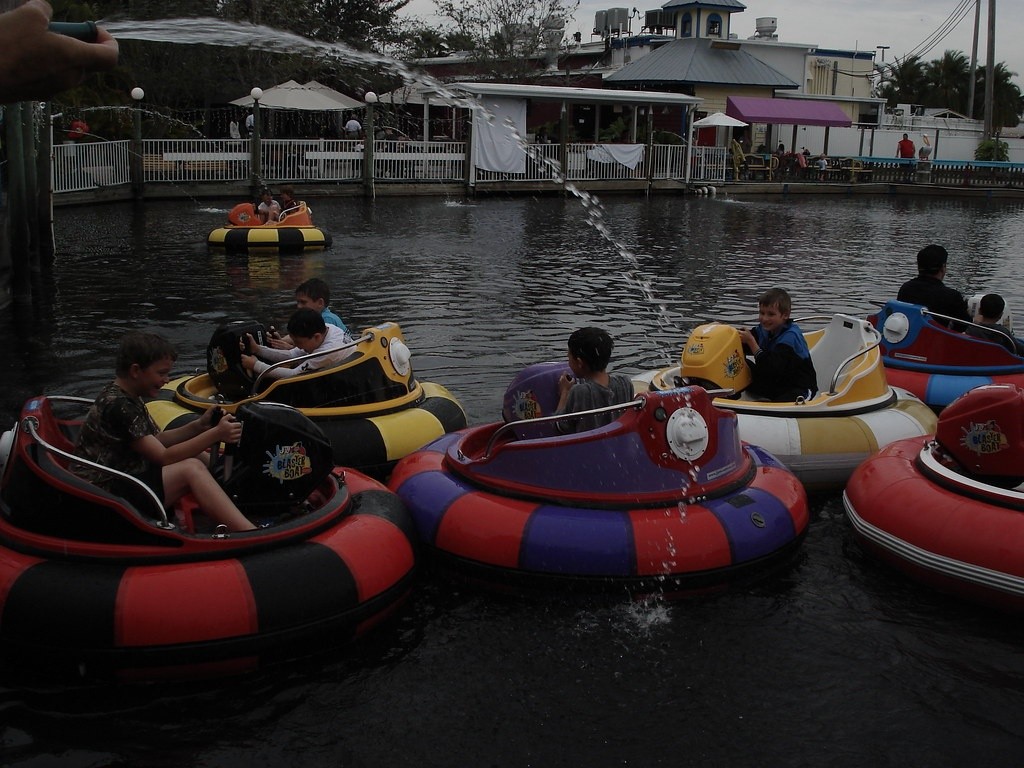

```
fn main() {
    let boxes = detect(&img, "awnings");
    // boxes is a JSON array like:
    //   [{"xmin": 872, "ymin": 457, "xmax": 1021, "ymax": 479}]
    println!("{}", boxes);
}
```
[{"xmin": 726, "ymin": 96, "xmax": 852, "ymax": 128}]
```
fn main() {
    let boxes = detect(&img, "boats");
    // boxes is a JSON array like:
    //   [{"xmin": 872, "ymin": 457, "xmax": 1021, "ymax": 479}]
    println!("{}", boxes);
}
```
[
  {"xmin": 1, "ymin": 373, "xmax": 426, "ymax": 687},
  {"xmin": 207, "ymin": 203, "xmax": 339, "ymax": 258},
  {"xmin": 829, "ymin": 384, "xmax": 1024, "ymax": 606},
  {"xmin": 843, "ymin": 296, "xmax": 1024, "ymax": 420},
  {"xmin": 389, "ymin": 389, "xmax": 810, "ymax": 608},
  {"xmin": 163, "ymin": 320, "xmax": 464, "ymax": 475},
  {"xmin": 626, "ymin": 313, "xmax": 947, "ymax": 512}
]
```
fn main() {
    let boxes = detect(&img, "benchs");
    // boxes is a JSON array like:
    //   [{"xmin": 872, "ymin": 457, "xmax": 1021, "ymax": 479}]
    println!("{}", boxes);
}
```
[
  {"xmin": 181, "ymin": 154, "xmax": 231, "ymax": 178},
  {"xmin": 838, "ymin": 158, "xmax": 873, "ymax": 181},
  {"xmin": 131, "ymin": 154, "xmax": 176, "ymax": 178},
  {"xmin": 698, "ymin": 153, "xmax": 774, "ymax": 182},
  {"xmin": 806, "ymin": 156, "xmax": 840, "ymax": 181}
]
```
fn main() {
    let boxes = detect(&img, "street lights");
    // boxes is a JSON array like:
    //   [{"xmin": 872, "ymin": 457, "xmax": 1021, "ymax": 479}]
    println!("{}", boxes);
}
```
[
  {"xmin": 250, "ymin": 86, "xmax": 262, "ymax": 187},
  {"xmin": 130, "ymin": 87, "xmax": 145, "ymax": 183},
  {"xmin": 362, "ymin": 91, "xmax": 378, "ymax": 186}
]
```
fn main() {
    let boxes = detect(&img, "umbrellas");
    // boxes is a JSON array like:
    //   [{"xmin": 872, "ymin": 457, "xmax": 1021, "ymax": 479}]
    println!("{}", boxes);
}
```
[
  {"xmin": 693, "ymin": 110, "xmax": 749, "ymax": 146},
  {"xmin": 228, "ymin": 80, "xmax": 353, "ymax": 137},
  {"xmin": 302, "ymin": 80, "xmax": 366, "ymax": 139},
  {"xmin": 376, "ymin": 80, "xmax": 472, "ymax": 108}
]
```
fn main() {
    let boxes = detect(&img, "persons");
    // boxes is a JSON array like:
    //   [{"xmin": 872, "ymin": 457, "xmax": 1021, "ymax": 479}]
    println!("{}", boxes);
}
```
[
  {"xmin": 273, "ymin": 187, "xmax": 298, "ymax": 222},
  {"xmin": 266, "ymin": 278, "xmax": 352, "ymax": 350},
  {"xmin": 801, "ymin": 147, "xmax": 810, "ymax": 155},
  {"xmin": 345, "ymin": 112, "xmax": 397, "ymax": 152},
  {"xmin": 897, "ymin": 244, "xmax": 973, "ymax": 334},
  {"xmin": 554, "ymin": 327, "xmax": 634, "ymax": 433},
  {"xmin": 68, "ymin": 333, "xmax": 291, "ymax": 532},
  {"xmin": 965, "ymin": 293, "xmax": 1024, "ymax": 358},
  {"xmin": 256, "ymin": 189, "xmax": 281, "ymax": 225},
  {"xmin": 815, "ymin": 153, "xmax": 828, "ymax": 180},
  {"xmin": 239, "ymin": 307, "xmax": 358, "ymax": 380},
  {"xmin": 230, "ymin": 107, "xmax": 260, "ymax": 138},
  {"xmin": 896, "ymin": 133, "xmax": 915, "ymax": 167},
  {"xmin": 535, "ymin": 127, "xmax": 549, "ymax": 143},
  {"xmin": 737, "ymin": 288, "xmax": 819, "ymax": 402},
  {"xmin": 776, "ymin": 143, "xmax": 784, "ymax": 157}
]
